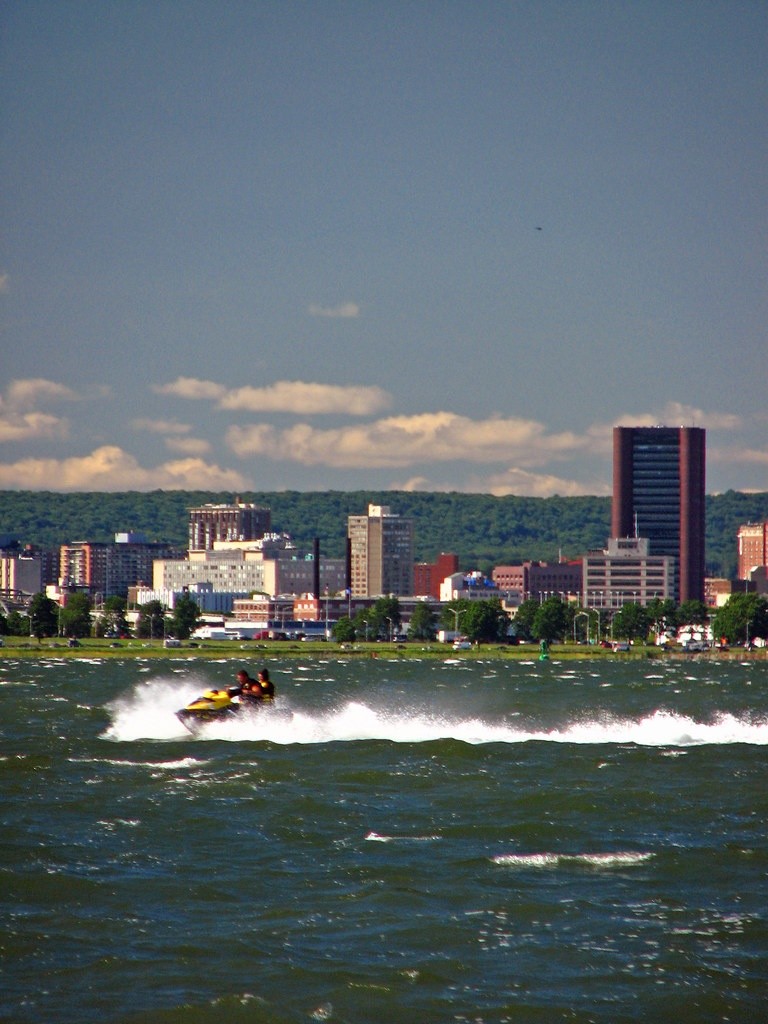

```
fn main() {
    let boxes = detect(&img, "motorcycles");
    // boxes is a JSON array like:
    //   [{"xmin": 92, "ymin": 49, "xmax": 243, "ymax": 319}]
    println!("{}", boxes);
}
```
[{"xmin": 175, "ymin": 684, "xmax": 293, "ymax": 736}]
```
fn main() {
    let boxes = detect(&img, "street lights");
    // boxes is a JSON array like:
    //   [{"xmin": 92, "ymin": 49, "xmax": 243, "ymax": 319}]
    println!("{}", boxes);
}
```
[
  {"xmin": 610, "ymin": 611, "xmax": 622, "ymax": 639},
  {"xmin": 573, "ymin": 614, "xmax": 582, "ymax": 644},
  {"xmin": 363, "ymin": 620, "xmax": 368, "ymax": 641},
  {"xmin": 145, "ymin": 614, "xmax": 155, "ymax": 640},
  {"xmin": 449, "ymin": 608, "xmax": 467, "ymax": 638},
  {"xmin": 386, "ymin": 617, "xmax": 399, "ymax": 643},
  {"xmin": 593, "ymin": 609, "xmax": 601, "ymax": 643},
  {"xmin": 282, "ymin": 605, "xmax": 293, "ymax": 629},
  {"xmin": 746, "ymin": 620, "xmax": 754, "ymax": 646},
  {"xmin": 579, "ymin": 612, "xmax": 589, "ymax": 645}
]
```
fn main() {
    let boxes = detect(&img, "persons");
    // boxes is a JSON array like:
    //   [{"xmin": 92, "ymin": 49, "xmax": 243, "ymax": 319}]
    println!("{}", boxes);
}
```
[
  {"xmin": 225, "ymin": 669, "xmax": 260, "ymax": 699},
  {"xmin": 254, "ymin": 670, "xmax": 275, "ymax": 699}
]
